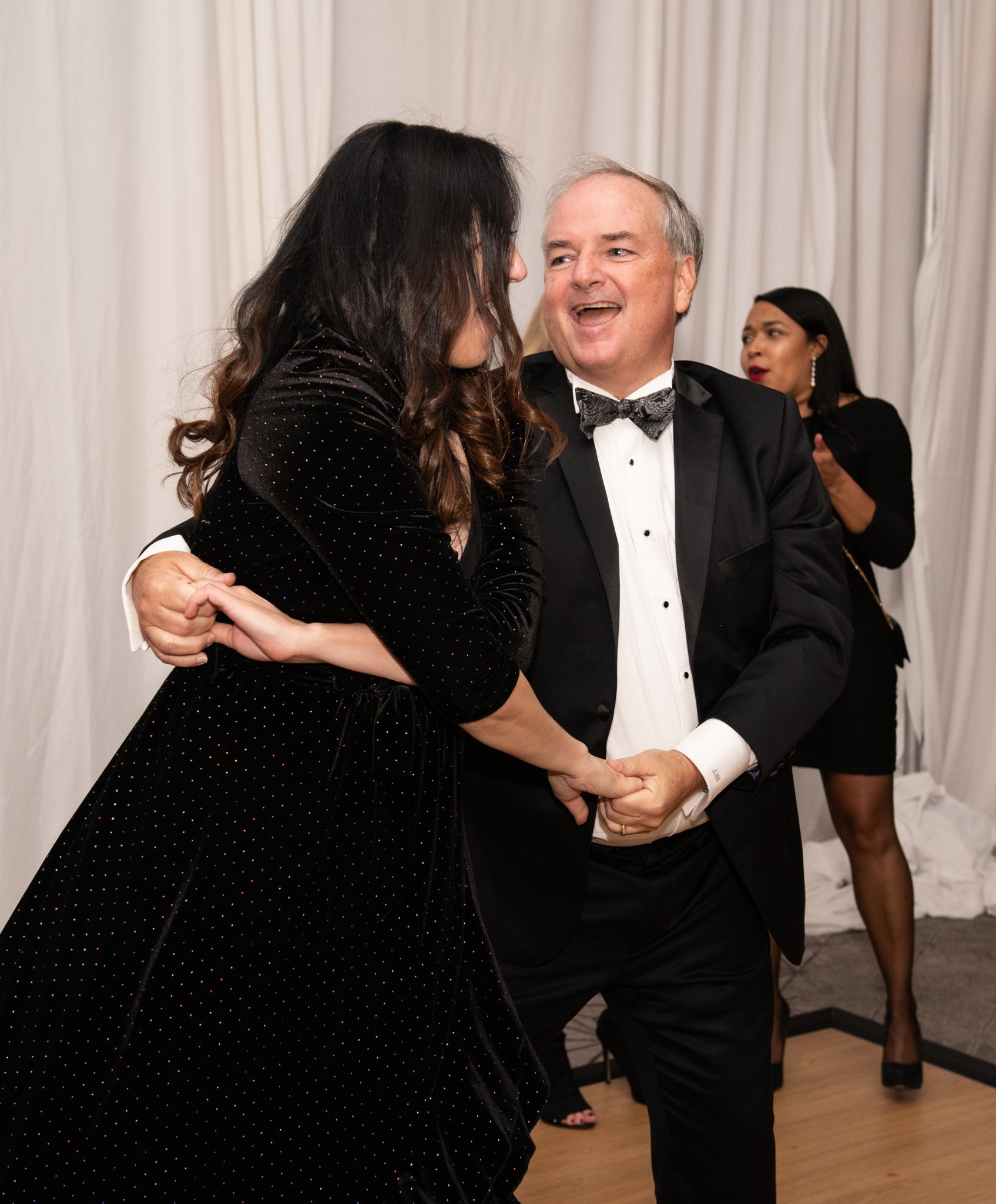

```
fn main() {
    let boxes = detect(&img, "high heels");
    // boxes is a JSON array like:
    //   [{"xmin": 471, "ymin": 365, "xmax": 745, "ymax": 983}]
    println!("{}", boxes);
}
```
[
  {"xmin": 882, "ymin": 1008, "xmax": 923, "ymax": 1092},
  {"xmin": 594, "ymin": 1011, "xmax": 647, "ymax": 1108},
  {"xmin": 770, "ymin": 997, "xmax": 791, "ymax": 1092},
  {"xmin": 532, "ymin": 1032, "xmax": 597, "ymax": 1130}
]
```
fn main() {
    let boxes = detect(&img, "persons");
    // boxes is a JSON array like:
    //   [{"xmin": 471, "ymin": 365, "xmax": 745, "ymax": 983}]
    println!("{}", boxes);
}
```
[
  {"xmin": 0, "ymin": 120, "xmax": 642, "ymax": 1204},
  {"xmin": 505, "ymin": 287, "xmax": 923, "ymax": 1131},
  {"xmin": 122, "ymin": 153, "xmax": 857, "ymax": 1204}
]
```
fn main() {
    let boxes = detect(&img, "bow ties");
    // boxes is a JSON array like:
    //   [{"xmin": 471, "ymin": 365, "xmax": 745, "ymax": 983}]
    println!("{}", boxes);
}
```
[{"xmin": 573, "ymin": 382, "xmax": 678, "ymax": 441}]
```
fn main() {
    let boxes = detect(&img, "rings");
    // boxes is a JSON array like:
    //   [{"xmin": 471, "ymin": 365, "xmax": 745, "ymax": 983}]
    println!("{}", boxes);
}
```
[{"xmin": 620, "ymin": 825, "xmax": 627, "ymax": 836}]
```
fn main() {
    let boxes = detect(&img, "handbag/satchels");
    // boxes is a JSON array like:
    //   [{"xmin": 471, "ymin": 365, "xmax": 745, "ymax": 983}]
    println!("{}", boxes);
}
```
[{"xmin": 885, "ymin": 612, "xmax": 910, "ymax": 668}]
